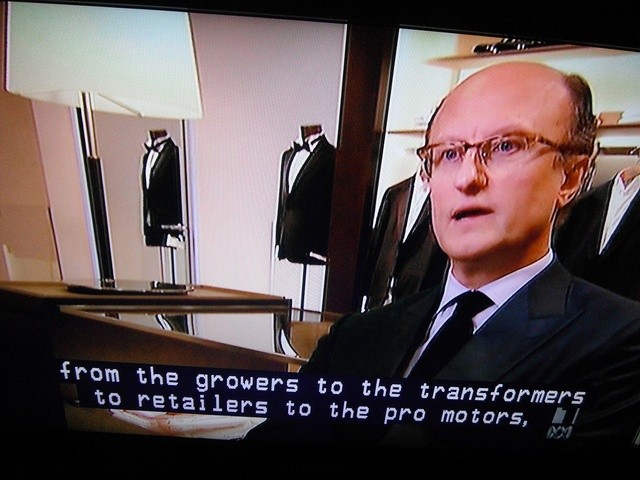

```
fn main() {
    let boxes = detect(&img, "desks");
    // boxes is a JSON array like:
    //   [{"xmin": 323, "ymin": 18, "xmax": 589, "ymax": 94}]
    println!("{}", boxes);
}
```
[{"xmin": 1, "ymin": 279, "xmax": 293, "ymax": 356}]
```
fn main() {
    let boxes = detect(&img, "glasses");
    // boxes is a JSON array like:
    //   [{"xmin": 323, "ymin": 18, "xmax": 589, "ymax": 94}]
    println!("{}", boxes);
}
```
[{"xmin": 415, "ymin": 131, "xmax": 570, "ymax": 174}]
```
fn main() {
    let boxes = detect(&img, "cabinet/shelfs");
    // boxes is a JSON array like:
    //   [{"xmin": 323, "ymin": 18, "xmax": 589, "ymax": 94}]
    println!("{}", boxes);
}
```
[{"xmin": 389, "ymin": 34, "xmax": 639, "ymax": 136}]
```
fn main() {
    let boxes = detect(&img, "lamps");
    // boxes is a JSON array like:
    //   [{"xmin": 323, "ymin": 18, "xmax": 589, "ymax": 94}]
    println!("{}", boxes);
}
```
[{"xmin": 6, "ymin": 2, "xmax": 201, "ymax": 318}]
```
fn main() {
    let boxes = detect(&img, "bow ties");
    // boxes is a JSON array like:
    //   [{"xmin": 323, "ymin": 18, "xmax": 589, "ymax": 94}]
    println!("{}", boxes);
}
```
[
  {"xmin": 144, "ymin": 139, "xmax": 167, "ymax": 152},
  {"xmin": 294, "ymin": 135, "xmax": 322, "ymax": 152}
]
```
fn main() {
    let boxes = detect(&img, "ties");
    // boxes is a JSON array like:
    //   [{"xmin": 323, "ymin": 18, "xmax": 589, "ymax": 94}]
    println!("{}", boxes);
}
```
[{"xmin": 406, "ymin": 291, "xmax": 495, "ymax": 380}]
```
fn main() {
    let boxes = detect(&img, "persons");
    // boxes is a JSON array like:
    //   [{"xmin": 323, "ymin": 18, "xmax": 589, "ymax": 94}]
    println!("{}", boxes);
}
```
[
  {"xmin": 244, "ymin": 62, "xmax": 639, "ymax": 441},
  {"xmin": 275, "ymin": 123, "xmax": 335, "ymax": 265},
  {"xmin": 141, "ymin": 130, "xmax": 185, "ymax": 248}
]
